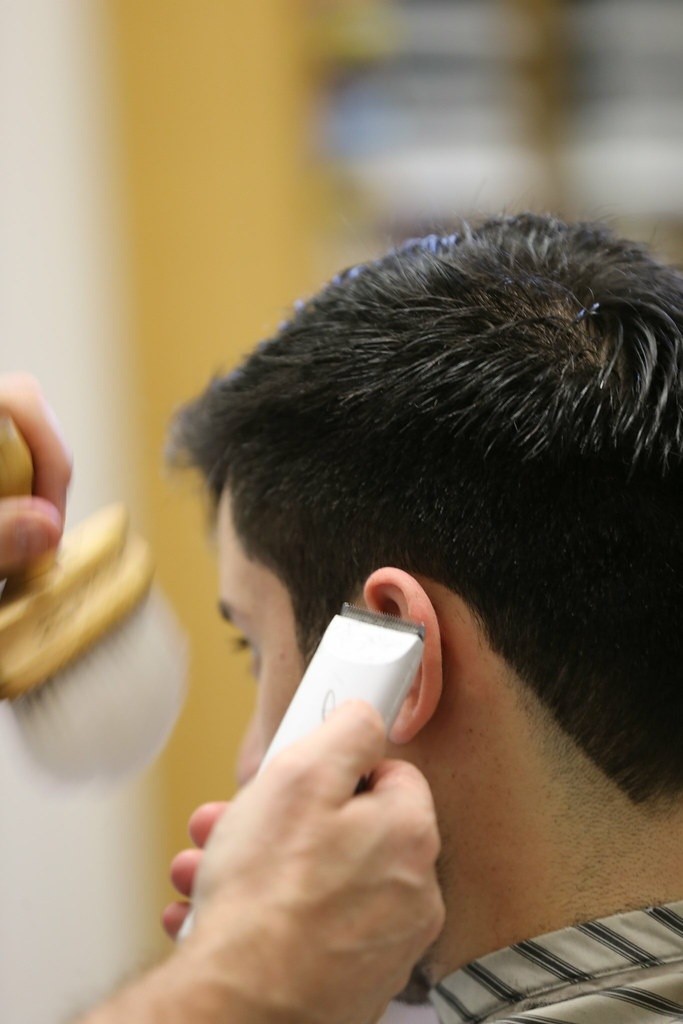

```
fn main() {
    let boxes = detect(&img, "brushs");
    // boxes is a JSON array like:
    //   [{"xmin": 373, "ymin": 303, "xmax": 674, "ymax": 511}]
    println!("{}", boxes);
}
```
[{"xmin": 0, "ymin": 406, "xmax": 190, "ymax": 791}]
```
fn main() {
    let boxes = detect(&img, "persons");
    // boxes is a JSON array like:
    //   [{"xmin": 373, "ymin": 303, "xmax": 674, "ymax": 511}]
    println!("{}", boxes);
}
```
[
  {"xmin": 174, "ymin": 214, "xmax": 683, "ymax": 1024},
  {"xmin": 0, "ymin": 372, "xmax": 445, "ymax": 1024}
]
